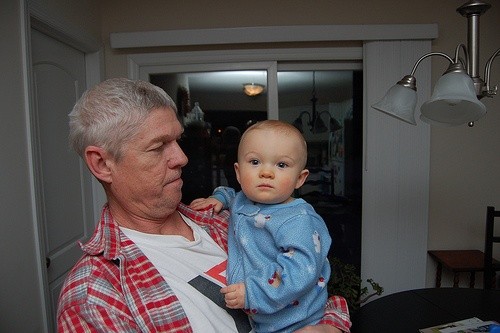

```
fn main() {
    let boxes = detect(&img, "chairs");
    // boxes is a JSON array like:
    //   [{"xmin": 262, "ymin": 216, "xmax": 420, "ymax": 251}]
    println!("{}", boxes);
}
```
[{"xmin": 484, "ymin": 206, "xmax": 500, "ymax": 289}]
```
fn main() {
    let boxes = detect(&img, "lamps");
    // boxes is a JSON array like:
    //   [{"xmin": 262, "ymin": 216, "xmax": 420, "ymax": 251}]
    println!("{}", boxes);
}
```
[
  {"xmin": 372, "ymin": 0, "xmax": 500, "ymax": 127},
  {"xmin": 292, "ymin": 71, "xmax": 341, "ymax": 136}
]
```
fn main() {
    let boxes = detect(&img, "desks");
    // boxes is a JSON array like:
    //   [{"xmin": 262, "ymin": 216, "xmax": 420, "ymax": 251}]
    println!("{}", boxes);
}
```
[{"xmin": 349, "ymin": 288, "xmax": 500, "ymax": 333}]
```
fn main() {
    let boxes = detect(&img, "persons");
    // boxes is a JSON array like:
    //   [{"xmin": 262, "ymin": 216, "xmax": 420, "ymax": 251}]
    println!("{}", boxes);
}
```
[
  {"xmin": 57, "ymin": 80, "xmax": 352, "ymax": 333},
  {"xmin": 190, "ymin": 120, "xmax": 331, "ymax": 333}
]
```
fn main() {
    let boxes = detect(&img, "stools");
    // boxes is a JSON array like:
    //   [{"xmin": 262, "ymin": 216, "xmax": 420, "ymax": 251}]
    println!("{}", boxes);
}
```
[{"xmin": 428, "ymin": 249, "xmax": 499, "ymax": 287}]
